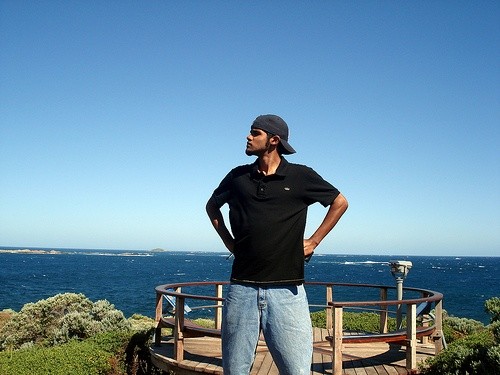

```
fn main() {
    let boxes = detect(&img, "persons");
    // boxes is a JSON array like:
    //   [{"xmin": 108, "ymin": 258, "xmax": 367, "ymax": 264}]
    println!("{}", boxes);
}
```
[{"xmin": 206, "ymin": 114, "xmax": 349, "ymax": 375}]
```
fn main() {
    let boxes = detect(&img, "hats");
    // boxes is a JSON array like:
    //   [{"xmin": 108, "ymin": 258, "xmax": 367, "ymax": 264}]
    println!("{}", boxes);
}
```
[{"xmin": 251, "ymin": 115, "xmax": 296, "ymax": 154}]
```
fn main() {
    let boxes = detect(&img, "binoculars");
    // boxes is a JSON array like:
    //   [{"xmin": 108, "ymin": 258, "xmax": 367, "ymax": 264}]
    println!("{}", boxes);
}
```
[{"xmin": 388, "ymin": 260, "xmax": 413, "ymax": 277}]
